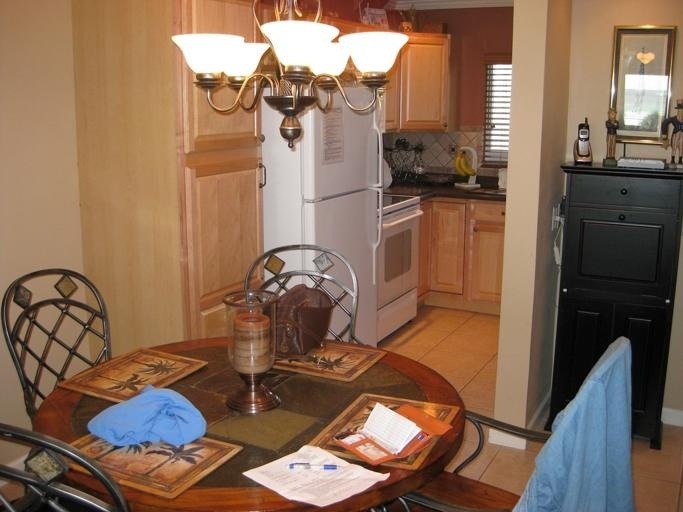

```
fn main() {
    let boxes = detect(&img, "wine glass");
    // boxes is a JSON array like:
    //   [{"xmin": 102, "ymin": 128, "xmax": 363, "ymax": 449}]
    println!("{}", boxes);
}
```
[{"xmin": 221, "ymin": 288, "xmax": 282, "ymax": 416}]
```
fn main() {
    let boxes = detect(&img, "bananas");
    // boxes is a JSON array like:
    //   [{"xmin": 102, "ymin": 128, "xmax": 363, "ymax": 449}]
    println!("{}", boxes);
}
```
[{"xmin": 455, "ymin": 150, "xmax": 475, "ymax": 176}]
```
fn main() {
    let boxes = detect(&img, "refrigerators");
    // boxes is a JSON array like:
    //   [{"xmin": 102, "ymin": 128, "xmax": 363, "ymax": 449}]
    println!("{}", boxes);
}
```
[{"xmin": 261, "ymin": 85, "xmax": 384, "ymax": 349}]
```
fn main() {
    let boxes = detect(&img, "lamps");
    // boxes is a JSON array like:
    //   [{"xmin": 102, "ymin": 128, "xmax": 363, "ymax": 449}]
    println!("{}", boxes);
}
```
[{"xmin": 170, "ymin": 1, "xmax": 410, "ymax": 147}]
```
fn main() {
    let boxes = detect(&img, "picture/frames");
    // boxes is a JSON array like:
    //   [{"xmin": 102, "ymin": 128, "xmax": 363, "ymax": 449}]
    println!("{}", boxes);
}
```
[{"xmin": 610, "ymin": 26, "xmax": 678, "ymax": 144}]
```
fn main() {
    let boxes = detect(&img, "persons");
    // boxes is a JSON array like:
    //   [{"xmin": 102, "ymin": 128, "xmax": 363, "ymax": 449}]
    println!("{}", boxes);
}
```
[
  {"xmin": 661, "ymin": 100, "xmax": 683, "ymax": 164},
  {"xmin": 606, "ymin": 109, "xmax": 619, "ymax": 157}
]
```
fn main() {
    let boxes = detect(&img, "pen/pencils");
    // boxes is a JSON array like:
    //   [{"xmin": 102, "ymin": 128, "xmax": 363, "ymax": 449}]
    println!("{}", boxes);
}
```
[{"xmin": 288, "ymin": 462, "xmax": 343, "ymax": 469}]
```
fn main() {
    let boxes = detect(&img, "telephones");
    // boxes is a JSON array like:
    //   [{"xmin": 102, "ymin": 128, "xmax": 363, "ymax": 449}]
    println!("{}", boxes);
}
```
[{"xmin": 573, "ymin": 117, "xmax": 592, "ymax": 165}]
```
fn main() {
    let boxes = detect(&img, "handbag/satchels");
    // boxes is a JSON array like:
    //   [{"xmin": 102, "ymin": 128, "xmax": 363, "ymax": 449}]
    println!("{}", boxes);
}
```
[{"xmin": 263, "ymin": 283, "xmax": 334, "ymax": 360}]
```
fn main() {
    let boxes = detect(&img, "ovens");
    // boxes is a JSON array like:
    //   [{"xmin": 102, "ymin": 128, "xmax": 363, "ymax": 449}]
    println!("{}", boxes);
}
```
[{"xmin": 370, "ymin": 202, "xmax": 424, "ymax": 343}]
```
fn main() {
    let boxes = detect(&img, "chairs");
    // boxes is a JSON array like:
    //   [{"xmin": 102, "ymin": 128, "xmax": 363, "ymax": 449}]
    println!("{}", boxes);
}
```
[
  {"xmin": 3, "ymin": 268, "xmax": 111, "ymax": 426},
  {"xmin": 368, "ymin": 417, "xmax": 524, "ymax": 511},
  {"xmin": 245, "ymin": 246, "xmax": 363, "ymax": 345},
  {"xmin": 1, "ymin": 424, "xmax": 128, "ymax": 511}
]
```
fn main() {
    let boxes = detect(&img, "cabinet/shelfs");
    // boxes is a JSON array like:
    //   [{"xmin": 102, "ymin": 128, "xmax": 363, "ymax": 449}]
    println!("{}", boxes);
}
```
[
  {"xmin": 386, "ymin": 43, "xmax": 449, "ymax": 133},
  {"xmin": 72, "ymin": 1, "xmax": 265, "ymax": 363},
  {"xmin": 420, "ymin": 195, "xmax": 505, "ymax": 317},
  {"xmin": 545, "ymin": 161, "xmax": 683, "ymax": 451}
]
